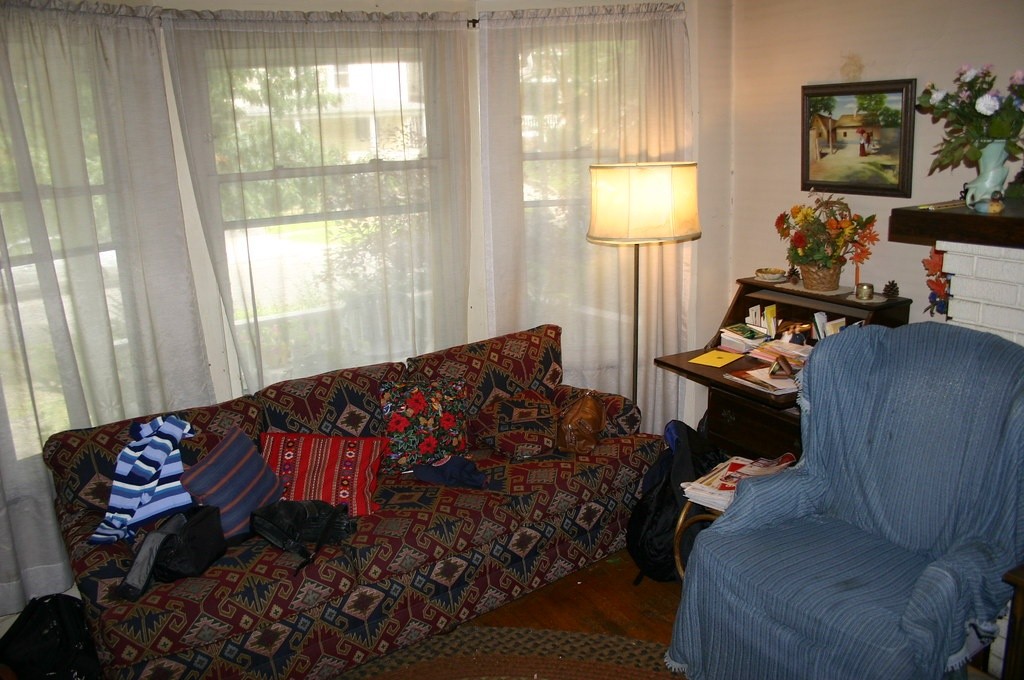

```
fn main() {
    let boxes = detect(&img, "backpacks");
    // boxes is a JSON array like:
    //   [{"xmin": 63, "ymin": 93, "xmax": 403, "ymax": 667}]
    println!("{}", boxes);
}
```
[{"xmin": 626, "ymin": 420, "xmax": 727, "ymax": 588}]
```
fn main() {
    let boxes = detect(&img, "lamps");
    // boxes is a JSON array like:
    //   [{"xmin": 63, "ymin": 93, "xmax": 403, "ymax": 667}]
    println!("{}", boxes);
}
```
[{"xmin": 585, "ymin": 162, "xmax": 704, "ymax": 404}]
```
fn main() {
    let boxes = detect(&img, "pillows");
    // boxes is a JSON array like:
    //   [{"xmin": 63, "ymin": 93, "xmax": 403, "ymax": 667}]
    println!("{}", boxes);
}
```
[
  {"xmin": 178, "ymin": 425, "xmax": 287, "ymax": 548},
  {"xmin": 376, "ymin": 378, "xmax": 469, "ymax": 478},
  {"xmin": 259, "ymin": 431, "xmax": 390, "ymax": 516},
  {"xmin": 465, "ymin": 388, "xmax": 561, "ymax": 464}
]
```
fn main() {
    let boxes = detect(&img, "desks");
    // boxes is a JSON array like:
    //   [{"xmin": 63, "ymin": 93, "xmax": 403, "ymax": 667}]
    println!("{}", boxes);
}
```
[{"xmin": 1000, "ymin": 564, "xmax": 1024, "ymax": 680}]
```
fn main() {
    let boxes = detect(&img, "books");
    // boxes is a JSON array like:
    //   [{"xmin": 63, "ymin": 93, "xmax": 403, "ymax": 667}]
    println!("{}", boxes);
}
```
[
  {"xmin": 723, "ymin": 363, "xmax": 802, "ymax": 395},
  {"xmin": 680, "ymin": 453, "xmax": 796, "ymax": 513}
]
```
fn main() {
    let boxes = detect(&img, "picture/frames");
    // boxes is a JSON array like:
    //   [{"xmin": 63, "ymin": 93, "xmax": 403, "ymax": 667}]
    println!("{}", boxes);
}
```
[{"xmin": 799, "ymin": 78, "xmax": 916, "ymax": 199}]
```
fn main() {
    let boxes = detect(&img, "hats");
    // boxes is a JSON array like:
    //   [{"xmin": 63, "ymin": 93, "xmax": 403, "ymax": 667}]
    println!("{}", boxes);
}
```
[{"xmin": 411, "ymin": 455, "xmax": 485, "ymax": 492}]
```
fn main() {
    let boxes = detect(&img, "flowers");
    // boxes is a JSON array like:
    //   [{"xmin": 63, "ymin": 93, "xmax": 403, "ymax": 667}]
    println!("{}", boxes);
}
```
[
  {"xmin": 922, "ymin": 246, "xmax": 955, "ymax": 317},
  {"xmin": 918, "ymin": 64, "xmax": 1024, "ymax": 176},
  {"xmin": 773, "ymin": 187, "xmax": 880, "ymax": 274}
]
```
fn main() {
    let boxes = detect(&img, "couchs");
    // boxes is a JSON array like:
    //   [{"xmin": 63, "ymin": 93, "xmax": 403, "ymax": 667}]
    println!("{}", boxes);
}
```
[{"xmin": 41, "ymin": 324, "xmax": 665, "ymax": 680}]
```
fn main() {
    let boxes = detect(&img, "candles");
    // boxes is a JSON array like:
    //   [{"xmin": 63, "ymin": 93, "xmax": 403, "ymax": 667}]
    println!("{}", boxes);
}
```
[{"xmin": 855, "ymin": 263, "xmax": 860, "ymax": 286}]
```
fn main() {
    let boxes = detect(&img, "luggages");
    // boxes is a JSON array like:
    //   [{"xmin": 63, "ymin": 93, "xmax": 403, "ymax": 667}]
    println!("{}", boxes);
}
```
[{"xmin": 0, "ymin": 593, "xmax": 105, "ymax": 680}]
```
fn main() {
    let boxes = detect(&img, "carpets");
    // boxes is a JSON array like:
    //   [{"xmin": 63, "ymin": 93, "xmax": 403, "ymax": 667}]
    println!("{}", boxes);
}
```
[{"xmin": 331, "ymin": 625, "xmax": 689, "ymax": 680}]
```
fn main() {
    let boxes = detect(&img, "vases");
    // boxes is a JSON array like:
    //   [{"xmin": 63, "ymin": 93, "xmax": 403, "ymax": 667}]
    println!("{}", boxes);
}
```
[
  {"xmin": 963, "ymin": 138, "xmax": 1010, "ymax": 210},
  {"xmin": 798, "ymin": 263, "xmax": 840, "ymax": 293}
]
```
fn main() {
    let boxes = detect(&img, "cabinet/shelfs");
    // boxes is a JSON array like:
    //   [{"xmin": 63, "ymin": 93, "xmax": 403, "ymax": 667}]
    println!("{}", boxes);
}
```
[{"xmin": 655, "ymin": 276, "xmax": 914, "ymax": 465}]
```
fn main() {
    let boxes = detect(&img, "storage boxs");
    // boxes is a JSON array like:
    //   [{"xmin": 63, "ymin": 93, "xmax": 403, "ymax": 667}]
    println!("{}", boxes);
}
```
[{"xmin": 720, "ymin": 333, "xmax": 751, "ymax": 351}]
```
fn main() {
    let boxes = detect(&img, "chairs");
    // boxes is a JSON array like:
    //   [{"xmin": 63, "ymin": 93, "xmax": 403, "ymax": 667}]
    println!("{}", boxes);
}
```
[{"xmin": 663, "ymin": 320, "xmax": 1024, "ymax": 680}]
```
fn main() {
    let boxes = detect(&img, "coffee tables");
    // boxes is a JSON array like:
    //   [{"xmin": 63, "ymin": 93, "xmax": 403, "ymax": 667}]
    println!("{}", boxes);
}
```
[{"xmin": 673, "ymin": 501, "xmax": 724, "ymax": 581}]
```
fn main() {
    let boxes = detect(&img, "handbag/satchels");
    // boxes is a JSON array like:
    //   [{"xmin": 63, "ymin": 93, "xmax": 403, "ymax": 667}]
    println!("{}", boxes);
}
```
[
  {"xmin": 251, "ymin": 498, "xmax": 349, "ymax": 580},
  {"xmin": 115, "ymin": 501, "xmax": 228, "ymax": 604}
]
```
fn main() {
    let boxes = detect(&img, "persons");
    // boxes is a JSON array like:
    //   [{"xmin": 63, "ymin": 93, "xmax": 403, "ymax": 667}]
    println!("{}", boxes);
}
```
[{"xmin": 780, "ymin": 324, "xmax": 806, "ymax": 346}]
